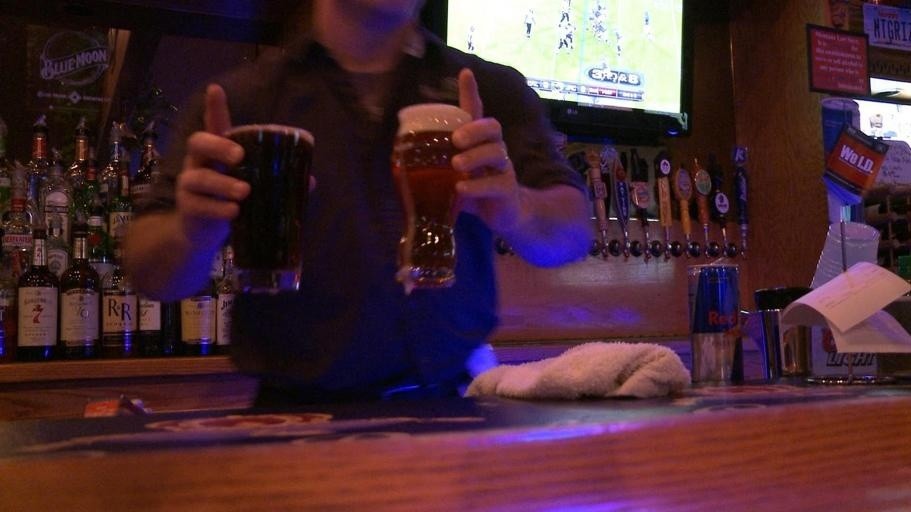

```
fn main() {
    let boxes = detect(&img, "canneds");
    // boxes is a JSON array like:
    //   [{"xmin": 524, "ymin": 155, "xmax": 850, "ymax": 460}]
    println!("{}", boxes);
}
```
[{"xmin": 689, "ymin": 264, "xmax": 742, "ymax": 386}]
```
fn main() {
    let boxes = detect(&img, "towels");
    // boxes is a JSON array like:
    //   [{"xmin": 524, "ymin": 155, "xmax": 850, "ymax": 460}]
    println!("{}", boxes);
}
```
[{"xmin": 465, "ymin": 337, "xmax": 697, "ymax": 401}]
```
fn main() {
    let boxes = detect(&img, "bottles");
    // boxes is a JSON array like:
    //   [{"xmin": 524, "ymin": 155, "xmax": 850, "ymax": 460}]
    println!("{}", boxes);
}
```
[{"xmin": 0, "ymin": 121, "xmax": 239, "ymax": 361}]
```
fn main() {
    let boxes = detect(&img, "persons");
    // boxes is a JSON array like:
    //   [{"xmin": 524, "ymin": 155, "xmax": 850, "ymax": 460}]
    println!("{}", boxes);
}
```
[
  {"xmin": 122, "ymin": 0, "xmax": 594, "ymax": 408},
  {"xmin": 555, "ymin": 0, "xmax": 651, "ymax": 58},
  {"xmin": 467, "ymin": 26, "xmax": 475, "ymax": 52},
  {"xmin": 523, "ymin": 8, "xmax": 536, "ymax": 38}
]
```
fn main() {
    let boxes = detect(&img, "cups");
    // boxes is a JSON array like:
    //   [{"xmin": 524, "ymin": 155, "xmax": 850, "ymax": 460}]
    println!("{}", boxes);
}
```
[
  {"xmin": 391, "ymin": 103, "xmax": 469, "ymax": 290},
  {"xmin": 810, "ymin": 220, "xmax": 880, "ymax": 288},
  {"xmin": 755, "ymin": 286, "xmax": 812, "ymax": 377},
  {"xmin": 222, "ymin": 123, "xmax": 316, "ymax": 295}
]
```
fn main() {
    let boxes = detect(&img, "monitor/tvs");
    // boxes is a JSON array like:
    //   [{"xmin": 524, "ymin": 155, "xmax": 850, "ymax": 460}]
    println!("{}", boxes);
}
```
[
  {"xmin": 2, "ymin": 1, "xmax": 282, "ymax": 46},
  {"xmin": 428, "ymin": 0, "xmax": 694, "ymax": 147}
]
